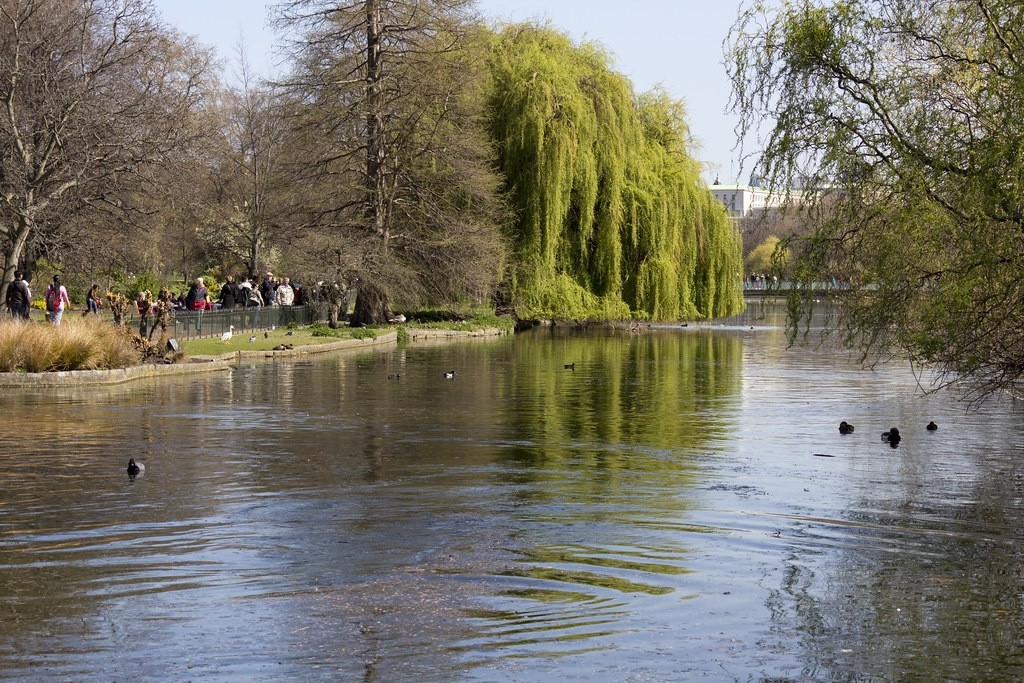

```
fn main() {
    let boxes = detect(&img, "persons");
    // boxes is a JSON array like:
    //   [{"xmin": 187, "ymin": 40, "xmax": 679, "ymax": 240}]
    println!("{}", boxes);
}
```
[
  {"xmin": 6, "ymin": 270, "xmax": 32, "ymax": 319},
  {"xmin": 137, "ymin": 273, "xmax": 303, "ymax": 333},
  {"xmin": 743, "ymin": 272, "xmax": 778, "ymax": 290},
  {"xmin": 45, "ymin": 275, "xmax": 70, "ymax": 325},
  {"xmin": 81, "ymin": 285, "xmax": 102, "ymax": 318}
]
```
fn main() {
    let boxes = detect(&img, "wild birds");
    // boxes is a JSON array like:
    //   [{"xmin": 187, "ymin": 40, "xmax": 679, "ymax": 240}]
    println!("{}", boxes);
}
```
[
  {"xmin": 388, "ymin": 373, "xmax": 401, "ymax": 381},
  {"xmin": 719, "ymin": 323, "xmax": 726, "ymax": 327},
  {"xmin": 926, "ymin": 420, "xmax": 938, "ymax": 431},
  {"xmin": 443, "ymin": 370, "xmax": 459, "ymax": 379},
  {"xmin": 126, "ymin": 457, "xmax": 146, "ymax": 473},
  {"xmin": 881, "ymin": 438, "xmax": 900, "ymax": 449},
  {"xmin": 681, "ymin": 324, "xmax": 688, "ymax": 328},
  {"xmin": 839, "ymin": 421, "xmax": 853, "ymax": 433},
  {"xmin": 563, "ymin": 362, "xmax": 576, "ymax": 369},
  {"xmin": 390, "ymin": 314, "xmax": 406, "ymax": 326},
  {"xmin": 882, "ymin": 426, "xmax": 901, "ymax": 438},
  {"xmin": 219, "ymin": 322, "xmax": 295, "ymax": 353},
  {"xmin": 750, "ymin": 325, "xmax": 755, "ymax": 330}
]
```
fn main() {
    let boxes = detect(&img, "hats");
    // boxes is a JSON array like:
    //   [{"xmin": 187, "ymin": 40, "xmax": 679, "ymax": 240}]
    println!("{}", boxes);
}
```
[{"xmin": 267, "ymin": 272, "xmax": 273, "ymax": 276}]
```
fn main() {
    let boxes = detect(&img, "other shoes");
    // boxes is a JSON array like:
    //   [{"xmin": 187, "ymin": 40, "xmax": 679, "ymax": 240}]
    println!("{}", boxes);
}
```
[{"xmin": 197, "ymin": 330, "xmax": 200, "ymax": 334}]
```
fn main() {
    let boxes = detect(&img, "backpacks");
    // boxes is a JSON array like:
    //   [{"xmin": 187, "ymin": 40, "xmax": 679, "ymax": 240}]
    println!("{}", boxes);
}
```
[{"xmin": 46, "ymin": 284, "xmax": 61, "ymax": 311}]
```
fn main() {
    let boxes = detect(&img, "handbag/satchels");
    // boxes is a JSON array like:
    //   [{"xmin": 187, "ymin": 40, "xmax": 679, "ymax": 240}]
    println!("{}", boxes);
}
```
[{"xmin": 249, "ymin": 290, "xmax": 260, "ymax": 306}]
